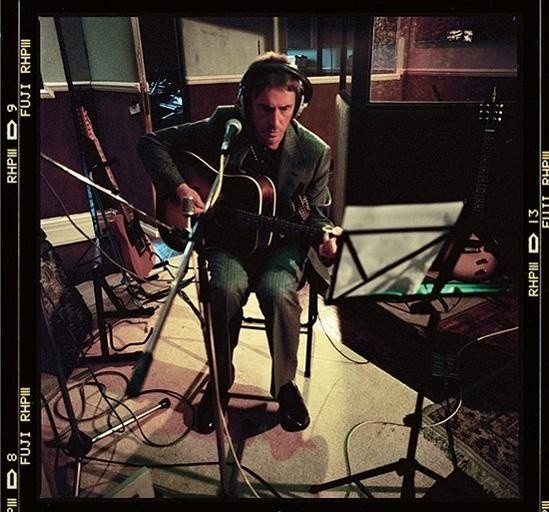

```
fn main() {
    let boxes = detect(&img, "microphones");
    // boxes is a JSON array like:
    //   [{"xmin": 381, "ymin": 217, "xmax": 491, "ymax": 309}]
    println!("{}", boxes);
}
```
[
  {"xmin": 181, "ymin": 196, "xmax": 195, "ymax": 216},
  {"xmin": 217, "ymin": 118, "xmax": 243, "ymax": 153}
]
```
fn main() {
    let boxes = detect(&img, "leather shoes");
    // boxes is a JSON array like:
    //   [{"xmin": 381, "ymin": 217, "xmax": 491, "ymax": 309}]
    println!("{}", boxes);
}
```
[
  {"xmin": 274, "ymin": 383, "xmax": 315, "ymax": 433},
  {"xmin": 195, "ymin": 377, "xmax": 226, "ymax": 434}
]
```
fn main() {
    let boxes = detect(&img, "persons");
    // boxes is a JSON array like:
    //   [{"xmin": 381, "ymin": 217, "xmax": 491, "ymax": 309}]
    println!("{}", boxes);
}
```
[{"xmin": 135, "ymin": 50, "xmax": 342, "ymax": 435}]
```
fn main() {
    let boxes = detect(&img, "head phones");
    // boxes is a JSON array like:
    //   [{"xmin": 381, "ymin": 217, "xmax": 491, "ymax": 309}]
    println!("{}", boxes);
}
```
[{"xmin": 234, "ymin": 63, "xmax": 314, "ymax": 119}]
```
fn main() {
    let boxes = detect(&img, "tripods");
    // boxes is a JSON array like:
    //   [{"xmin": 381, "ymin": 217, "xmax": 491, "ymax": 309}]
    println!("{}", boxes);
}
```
[
  {"xmin": 40, "ymin": 293, "xmax": 172, "ymax": 499},
  {"xmin": 309, "ymin": 309, "xmax": 447, "ymax": 506}
]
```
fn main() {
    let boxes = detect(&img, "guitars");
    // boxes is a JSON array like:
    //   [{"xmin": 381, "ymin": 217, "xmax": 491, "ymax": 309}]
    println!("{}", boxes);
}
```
[
  {"xmin": 74, "ymin": 106, "xmax": 157, "ymax": 281},
  {"xmin": 154, "ymin": 150, "xmax": 347, "ymax": 263}
]
